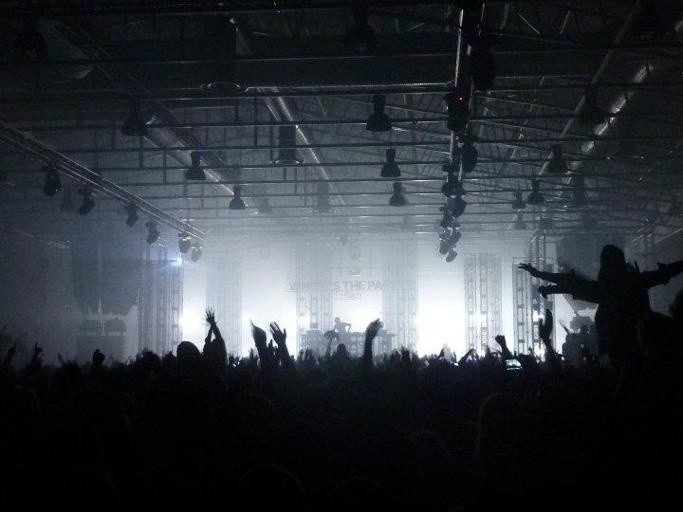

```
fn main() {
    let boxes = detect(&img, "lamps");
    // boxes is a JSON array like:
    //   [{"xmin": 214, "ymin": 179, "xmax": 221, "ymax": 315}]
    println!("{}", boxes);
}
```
[
  {"xmin": 46, "ymin": 161, "xmax": 61, "ymax": 196},
  {"xmin": 177, "ymin": 230, "xmax": 192, "ymax": 254},
  {"xmin": 147, "ymin": 220, "xmax": 160, "ymax": 244},
  {"xmin": 120, "ymin": 101, "xmax": 149, "ymax": 137},
  {"xmin": 124, "ymin": 202, "xmax": 140, "ymax": 228},
  {"xmin": 184, "ymin": 151, "xmax": 207, "ymax": 181},
  {"xmin": 438, "ymin": 14, "xmax": 607, "ymax": 263},
  {"xmin": 260, "ymin": 198, "xmax": 274, "ymax": 214},
  {"xmin": 364, "ymin": 95, "xmax": 393, "ymax": 132},
  {"xmin": 388, "ymin": 183, "xmax": 407, "ymax": 207},
  {"xmin": 229, "ymin": 186, "xmax": 246, "ymax": 210},
  {"xmin": 78, "ymin": 182, "xmax": 97, "ymax": 216},
  {"xmin": 190, "ymin": 242, "xmax": 203, "ymax": 261},
  {"xmin": 379, "ymin": 148, "xmax": 402, "ymax": 178}
]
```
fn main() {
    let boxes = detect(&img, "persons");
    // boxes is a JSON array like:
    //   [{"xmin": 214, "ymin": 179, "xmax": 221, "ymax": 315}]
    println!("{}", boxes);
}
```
[{"xmin": 1, "ymin": 244, "xmax": 683, "ymax": 512}]
